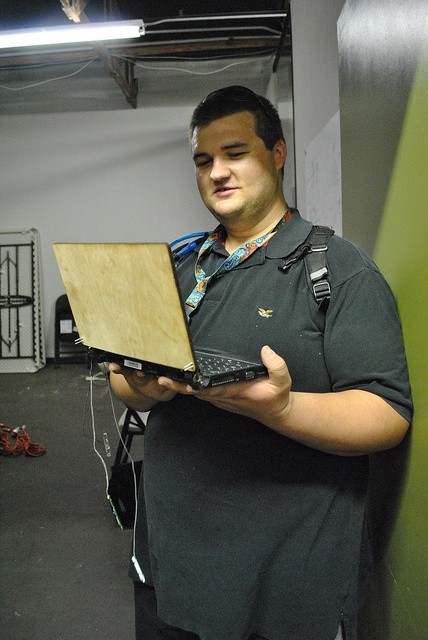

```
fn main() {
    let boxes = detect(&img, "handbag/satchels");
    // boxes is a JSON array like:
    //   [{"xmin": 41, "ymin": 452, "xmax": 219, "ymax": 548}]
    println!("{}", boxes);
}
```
[{"xmin": 111, "ymin": 460, "xmax": 143, "ymax": 528}]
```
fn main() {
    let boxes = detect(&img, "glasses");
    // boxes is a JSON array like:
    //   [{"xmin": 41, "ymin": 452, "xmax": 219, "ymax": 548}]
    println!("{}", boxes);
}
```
[{"xmin": 198, "ymin": 86, "xmax": 281, "ymax": 140}]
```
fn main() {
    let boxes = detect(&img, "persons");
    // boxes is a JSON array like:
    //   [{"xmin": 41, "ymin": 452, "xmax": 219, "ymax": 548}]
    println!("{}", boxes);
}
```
[{"xmin": 109, "ymin": 84, "xmax": 414, "ymax": 640}]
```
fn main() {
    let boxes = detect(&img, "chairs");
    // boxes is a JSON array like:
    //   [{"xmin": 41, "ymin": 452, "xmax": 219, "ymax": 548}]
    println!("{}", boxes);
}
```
[
  {"xmin": 114, "ymin": 408, "xmax": 150, "ymax": 465},
  {"xmin": 54, "ymin": 294, "xmax": 91, "ymax": 370}
]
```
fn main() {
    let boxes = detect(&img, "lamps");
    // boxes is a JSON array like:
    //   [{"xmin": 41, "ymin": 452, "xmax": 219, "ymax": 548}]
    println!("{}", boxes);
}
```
[{"xmin": 0, "ymin": 18, "xmax": 146, "ymax": 48}]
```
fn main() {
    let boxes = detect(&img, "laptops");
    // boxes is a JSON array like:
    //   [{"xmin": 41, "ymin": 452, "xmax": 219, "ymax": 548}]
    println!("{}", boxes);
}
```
[{"xmin": 52, "ymin": 241, "xmax": 270, "ymax": 393}]
